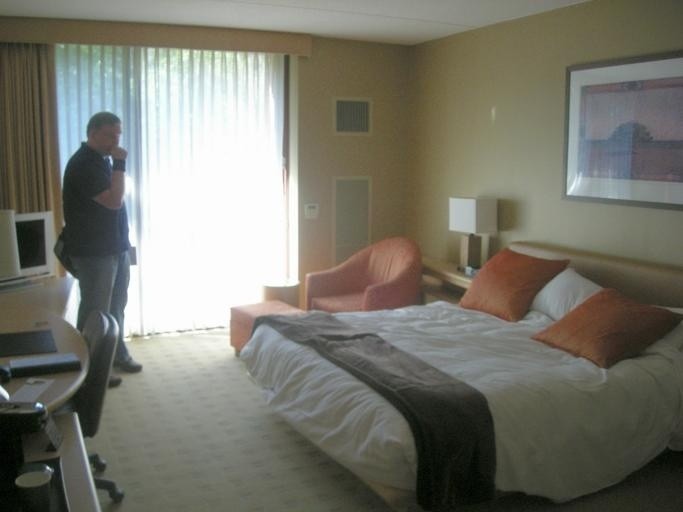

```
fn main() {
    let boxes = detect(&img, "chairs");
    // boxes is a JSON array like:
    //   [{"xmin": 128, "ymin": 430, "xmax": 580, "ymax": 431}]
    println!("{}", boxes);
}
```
[
  {"xmin": 304, "ymin": 236, "xmax": 421, "ymax": 312},
  {"xmin": 71, "ymin": 311, "xmax": 126, "ymax": 503}
]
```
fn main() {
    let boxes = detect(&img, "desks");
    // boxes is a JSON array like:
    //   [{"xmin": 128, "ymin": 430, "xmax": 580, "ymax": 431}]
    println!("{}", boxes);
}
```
[
  {"xmin": 0, "ymin": 276, "xmax": 90, "ymax": 411},
  {"xmin": 0, "ymin": 411, "xmax": 103, "ymax": 512}
]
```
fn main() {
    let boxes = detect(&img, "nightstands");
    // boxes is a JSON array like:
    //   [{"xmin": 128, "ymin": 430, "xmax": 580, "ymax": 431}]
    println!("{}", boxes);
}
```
[{"xmin": 421, "ymin": 263, "xmax": 472, "ymax": 304}]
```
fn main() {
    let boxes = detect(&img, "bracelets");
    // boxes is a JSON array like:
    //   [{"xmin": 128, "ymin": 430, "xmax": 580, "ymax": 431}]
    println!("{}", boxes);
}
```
[{"xmin": 112, "ymin": 158, "xmax": 125, "ymax": 171}]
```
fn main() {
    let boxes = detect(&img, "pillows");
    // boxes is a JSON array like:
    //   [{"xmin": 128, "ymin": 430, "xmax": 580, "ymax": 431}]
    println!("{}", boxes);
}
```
[{"xmin": 459, "ymin": 247, "xmax": 683, "ymax": 369}]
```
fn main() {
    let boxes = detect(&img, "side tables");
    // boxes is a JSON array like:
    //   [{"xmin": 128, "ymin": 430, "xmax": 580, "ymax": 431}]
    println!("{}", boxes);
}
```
[{"xmin": 260, "ymin": 278, "xmax": 300, "ymax": 309}]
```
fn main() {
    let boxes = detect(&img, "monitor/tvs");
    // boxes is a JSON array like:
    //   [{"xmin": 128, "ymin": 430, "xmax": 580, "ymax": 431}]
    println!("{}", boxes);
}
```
[{"xmin": 0, "ymin": 210, "xmax": 56, "ymax": 293}]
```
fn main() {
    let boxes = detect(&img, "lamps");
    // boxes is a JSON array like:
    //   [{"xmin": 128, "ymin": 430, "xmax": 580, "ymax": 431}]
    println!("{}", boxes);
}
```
[{"xmin": 449, "ymin": 195, "xmax": 498, "ymax": 271}]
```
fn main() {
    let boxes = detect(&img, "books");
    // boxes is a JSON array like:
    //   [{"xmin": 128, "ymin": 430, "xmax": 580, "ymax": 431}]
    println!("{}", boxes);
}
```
[{"xmin": 10, "ymin": 351, "xmax": 82, "ymax": 379}]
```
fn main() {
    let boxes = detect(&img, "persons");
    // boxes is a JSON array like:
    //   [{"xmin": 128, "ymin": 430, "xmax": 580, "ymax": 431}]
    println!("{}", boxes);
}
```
[{"xmin": 60, "ymin": 112, "xmax": 143, "ymax": 389}]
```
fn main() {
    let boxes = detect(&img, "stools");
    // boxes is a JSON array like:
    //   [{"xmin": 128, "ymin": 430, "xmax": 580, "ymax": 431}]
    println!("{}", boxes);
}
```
[{"xmin": 230, "ymin": 300, "xmax": 304, "ymax": 357}]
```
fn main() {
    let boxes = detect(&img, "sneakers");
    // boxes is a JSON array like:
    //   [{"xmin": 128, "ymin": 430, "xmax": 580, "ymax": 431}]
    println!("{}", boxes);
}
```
[
  {"xmin": 109, "ymin": 375, "xmax": 122, "ymax": 388},
  {"xmin": 114, "ymin": 358, "xmax": 142, "ymax": 371}
]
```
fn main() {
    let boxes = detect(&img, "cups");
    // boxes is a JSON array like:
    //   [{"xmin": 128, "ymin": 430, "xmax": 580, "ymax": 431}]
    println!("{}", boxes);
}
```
[{"xmin": 15, "ymin": 470, "xmax": 52, "ymax": 512}]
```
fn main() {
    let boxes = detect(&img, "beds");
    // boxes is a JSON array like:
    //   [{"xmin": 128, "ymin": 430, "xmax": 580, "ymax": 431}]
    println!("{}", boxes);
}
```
[{"xmin": 238, "ymin": 242, "xmax": 683, "ymax": 512}]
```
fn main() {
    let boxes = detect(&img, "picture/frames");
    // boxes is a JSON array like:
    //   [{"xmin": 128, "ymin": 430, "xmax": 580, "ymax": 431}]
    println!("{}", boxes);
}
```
[{"xmin": 561, "ymin": 50, "xmax": 683, "ymax": 211}]
